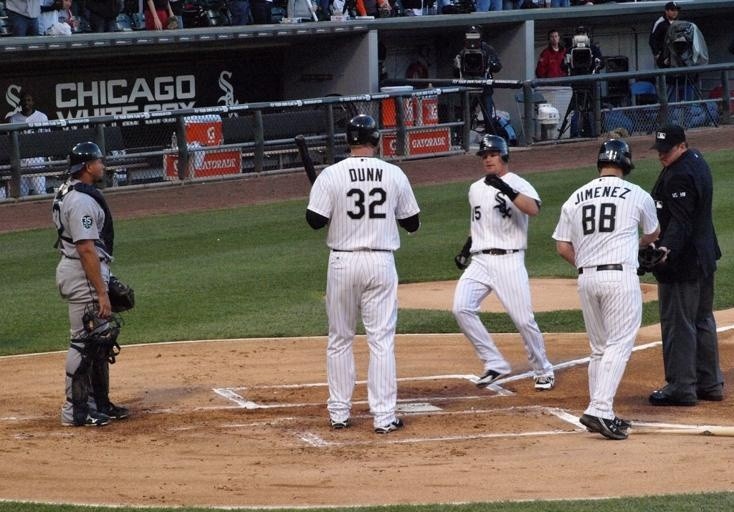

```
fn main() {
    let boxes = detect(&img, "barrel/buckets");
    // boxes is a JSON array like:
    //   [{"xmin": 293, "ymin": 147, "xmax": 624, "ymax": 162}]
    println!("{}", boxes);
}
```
[
  {"xmin": 537, "ymin": 103, "xmax": 560, "ymax": 140},
  {"xmin": 413, "ymin": 90, "xmax": 439, "ymax": 125},
  {"xmin": 381, "ymin": 86, "xmax": 413, "ymax": 126}
]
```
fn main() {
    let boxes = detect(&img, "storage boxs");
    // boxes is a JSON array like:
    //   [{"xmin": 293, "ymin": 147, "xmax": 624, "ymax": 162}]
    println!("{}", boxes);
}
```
[
  {"xmin": 163, "ymin": 114, "xmax": 243, "ymax": 180},
  {"xmin": 380, "ymin": 85, "xmax": 451, "ymax": 162}
]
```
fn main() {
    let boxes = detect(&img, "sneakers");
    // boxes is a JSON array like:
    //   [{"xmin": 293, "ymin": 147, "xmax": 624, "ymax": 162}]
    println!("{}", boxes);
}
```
[
  {"xmin": 330, "ymin": 418, "xmax": 352, "ymax": 430},
  {"xmin": 648, "ymin": 381, "xmax": 724, "ymax": 407},
  {"xmin": 374, "ymin": 417, "xmax": 404, "ymax": 435},
  {"xmin": 534, "ymin": 373, "xmax": 556, "ymax": 391},
  {"xmin": 62, "ymin": 402, "xmax": 130, "ymax": 427},
  {"xmin": 475, "ymin": 366, "xmax": 509, "ymax": 389},
  {"xmin": 579, "ymin": 413, "xmax": 630, "ymax": 441}
]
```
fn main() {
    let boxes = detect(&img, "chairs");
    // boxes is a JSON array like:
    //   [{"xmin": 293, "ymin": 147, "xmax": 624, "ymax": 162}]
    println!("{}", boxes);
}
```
[
  {"xmin": 630, "ymin": 82, "xmax": 659, "ymax": 135},
  {"xmin": 514, "ymin": 92, "xmax": 555, "ymax": 146}
]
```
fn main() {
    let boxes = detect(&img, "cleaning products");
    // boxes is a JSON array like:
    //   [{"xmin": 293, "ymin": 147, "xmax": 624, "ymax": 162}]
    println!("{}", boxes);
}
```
[{"xmin": 172, "ymin": 132, "xmax": 178, "ymax": 149}]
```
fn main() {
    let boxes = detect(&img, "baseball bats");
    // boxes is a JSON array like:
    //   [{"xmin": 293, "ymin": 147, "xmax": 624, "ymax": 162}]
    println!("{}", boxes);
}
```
[{"xmin": 295, "ymin": 133, "xmax": 317, "ymax": 183}]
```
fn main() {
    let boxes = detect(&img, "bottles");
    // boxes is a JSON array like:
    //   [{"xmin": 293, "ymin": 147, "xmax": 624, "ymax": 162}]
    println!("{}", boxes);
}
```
[
  {"xmin": 171, "ymin": 132, "xmax": 177, "ymax": 150},
  {"xmin": 112, "ymin": 173, "xmax": 119, "ymax": 191}
]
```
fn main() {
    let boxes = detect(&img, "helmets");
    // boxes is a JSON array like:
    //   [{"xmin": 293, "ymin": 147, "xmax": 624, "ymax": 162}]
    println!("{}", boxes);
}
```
[
  {"xmin": 597, "ymin": 138, "xmax": 634, "ymax": 176},
  {"xmin": 476, "ymin": 134, "xmax": 509, "ymax": 163},
  {"xmin": 69, "ymin": 142, "xmax": 104, "ymax": 173},
  {"xmin": 346, "ymin": 114, "xmax": 382, "ymax": 148}
]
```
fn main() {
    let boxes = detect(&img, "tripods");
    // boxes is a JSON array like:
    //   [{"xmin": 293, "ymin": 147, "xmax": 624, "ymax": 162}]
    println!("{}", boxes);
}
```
[
  {"xmin": 647, "ymin": 74, "xmax": 718, "ymax": 136},
  {"xmin": 556, "ymin": 82, "xmax": 609, "ymax": 138},
  {"xmin": 456, "ymin": 94, "xmax": 498, "ymax": 147}
]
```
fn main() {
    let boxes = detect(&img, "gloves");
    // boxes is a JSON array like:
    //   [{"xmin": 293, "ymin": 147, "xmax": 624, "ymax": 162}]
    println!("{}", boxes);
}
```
[
  {"xmin": 454, "ymin": 236, "xmax": 473, "ymax": 269},
  {"xmin": 483, "ymin": 173, "xmax": 519, "ymax": 201}
]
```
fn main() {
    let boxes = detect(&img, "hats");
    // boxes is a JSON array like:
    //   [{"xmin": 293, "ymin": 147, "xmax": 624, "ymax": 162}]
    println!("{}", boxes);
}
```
[
  {"xmin": 664, "ymin": 1, "xmax": 684, "ymax": 12},
  {"xmin": 648, "ymin": 124, "xmax": 686, "ymax": 154}
]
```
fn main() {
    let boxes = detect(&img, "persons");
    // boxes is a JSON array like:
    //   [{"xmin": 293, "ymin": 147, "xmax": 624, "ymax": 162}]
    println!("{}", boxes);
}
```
[
  {"xmin": 635, "ymin": 123, "xmax": 726, "ymax": 406},
  {"xmin": 561, "ymin": 26, "xmax": 605, "ymax": 138},
  {"xmin": 51, "ymin": 141, "xmax": 129, "ymax": 427},
  {"xmin": 535, "ymin": 28, "xmax": 569, "ymax": 88},
  {"xmin": 1, "ymin": 1, "xmax": 637, "ymax": 37},
  {"xmin": 551, "ymin": 139, "xmax": 662, "ymax": 440},
  {"xmin": 648, "ymin": 2, "xmax": 703, "ymax": 130},
  {"xmin": 451, "ymin": 132, "xmax": 557, "ymax": 390},
  {"xmin": 452, "ymin": 26, "xmax": 503, "ymax": 148},
  {"xmin": 306, "ymin": 112, "xmax": 421, "ymax": 433},
  {"xmin": 7, "ymin": 93, "xmax": 52, "ymax": 196}
]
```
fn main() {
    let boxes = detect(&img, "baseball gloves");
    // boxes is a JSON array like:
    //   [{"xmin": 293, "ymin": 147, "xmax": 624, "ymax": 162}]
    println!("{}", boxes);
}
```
[
  {"xmin": 637, "ymin": 243, "xmax": 667, "ymax": 276},
  {"xmin": 107, "ymin": 276, "xmax": 134, "ymax": 313}
]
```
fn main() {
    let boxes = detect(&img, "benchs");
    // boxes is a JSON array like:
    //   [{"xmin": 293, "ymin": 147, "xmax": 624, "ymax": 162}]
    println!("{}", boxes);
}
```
[{"xmin": 0, "ymin": 109, "xmax": 346, "ymax": 189}]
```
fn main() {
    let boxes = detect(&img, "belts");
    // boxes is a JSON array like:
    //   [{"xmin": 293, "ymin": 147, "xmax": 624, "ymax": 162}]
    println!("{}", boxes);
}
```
[
  {"xmin": 330, "ymin": 248, "xmax": 395, "ymax": 253},
  {"xmin": 472, "ymin": 247, "xmax": 521, "ymax": 256},
  {"xmin": 577, "ymin": 264, "xmax": 626, "ymax": 274}
]
